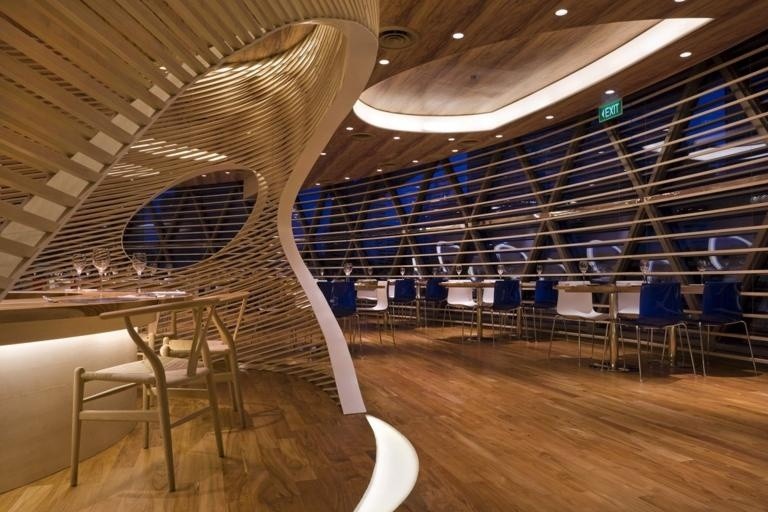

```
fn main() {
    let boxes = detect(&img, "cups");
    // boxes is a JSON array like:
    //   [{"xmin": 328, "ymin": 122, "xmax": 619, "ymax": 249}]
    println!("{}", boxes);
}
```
[{"xmin": 367, "ymin": 268, "xmax": 373, "ymax": 275}]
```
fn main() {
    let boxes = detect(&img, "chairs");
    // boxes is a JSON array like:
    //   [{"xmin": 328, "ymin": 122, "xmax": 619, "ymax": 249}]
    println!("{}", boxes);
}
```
[{"xmin": 68, "ymin": 291, "xmax": 249, "ymax": 493}]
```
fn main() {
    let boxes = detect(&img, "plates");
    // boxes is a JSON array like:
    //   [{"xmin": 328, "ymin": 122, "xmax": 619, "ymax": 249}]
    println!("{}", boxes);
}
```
[
  {"xmin": 147, "ymin": 291, "xmax": 187, "ymax": 298},
  {"xmin": 448, "ymin": 279, "xmax": 472, "ymax": 282},
  {"xmin": 483, "ymin": 279, "xmax": 504, "ymax": 283},
  {"xmin": 615, "ymin": 280, "xmax": 645, "ymax": 285},
  {"xmin": 558, "ymin": 280, "xmax": 591, "ymax": 285}
]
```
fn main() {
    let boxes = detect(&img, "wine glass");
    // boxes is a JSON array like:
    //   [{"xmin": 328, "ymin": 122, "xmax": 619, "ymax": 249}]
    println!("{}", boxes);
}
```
[
  {"xmin": 72, "ymin": 253, "xmax": 87, "ymax": 293},
  {"xmin": 579, "ymin": 260, "xmax": 588, "ymax": 282},
  {"xmin": 131, "ymin": 252, "xmax": 148, "ymax": 297},
  {"xmin": 497, "ymin": 264, "xmax": 504, "ymax": 281},
  {"xmin": 535, "ymin": 264, "xmax": 544, "ymax": 281},
  {"xmin": 400, "ymin": 267, "xmax": 405, "ymax": 280},
  {"xmin": 456, "ymin": 264, "xmax": 463, "ymax": 280},
  {"xmin": 343, "ymin": 262, "xmax": 353, "ymax": 282},
  {"xmin": 639, "ymin": 258, "xmax": 650, "ymax": 284},
  {"xmin": 696, "ymin": 256, "xmax": 709, "ymax": 285},
  {"xmin": 92, "ymin": 247, "xmax": 111, "ymax": 296}
]
